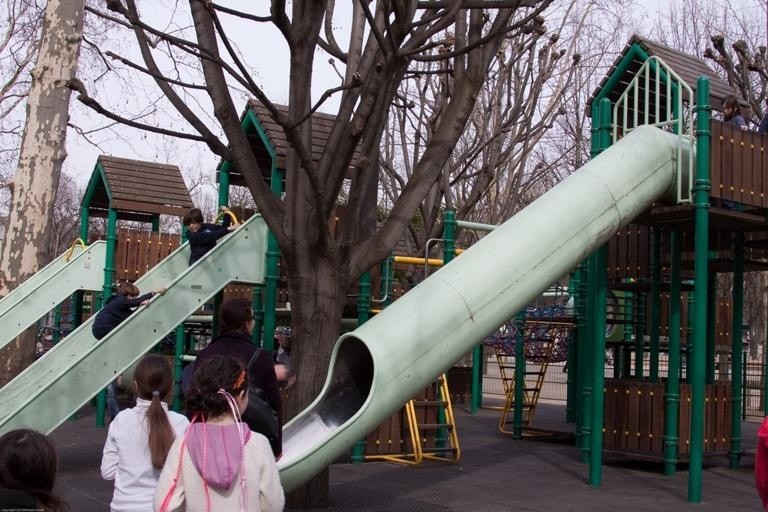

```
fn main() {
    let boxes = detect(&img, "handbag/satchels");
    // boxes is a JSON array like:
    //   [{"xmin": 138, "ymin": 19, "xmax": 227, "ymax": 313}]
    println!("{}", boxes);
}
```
[{"xmin": 241, "ymin": 347, "xmax": 282, "ymax": 457}]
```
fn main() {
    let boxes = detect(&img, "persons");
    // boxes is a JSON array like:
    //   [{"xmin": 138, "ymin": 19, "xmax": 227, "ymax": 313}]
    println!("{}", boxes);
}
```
[
  {"xmin": 182, "ymin": 205, "xmax": 240, "ymax": 266},
  {"xmin": 106, "ymin": 375, "xmax": 123, "ymax": 419},
  {"xmin": 163, "ymin": 345, "xmax": 172, "ymax": 354},
  {"xmin": 758, "ymin": 99, "xmax": 768, "ymax": 135},
  {"xmin": 155, "ymin": 356, "xmax": 286, "ymax": 512},
  {"xmin": 0, "ymin": 429, "xmax": 71, "ymax": 512},
  {"xmin": 184, "ymin": 343, "xmax": 196, "ymax": 356},
  {"xmin": 277, "ymin": 338, "xmax": 294, "ymax": 388},
  {"xmin": 192, "ymin": 298, "xmax": 283, "ymax": 462},
  {"xmin": 101, "ymin": 354, "xmax": 191, "ymax": 512},
  {"xmin": 720, "ymin": 94, "xmax": 745, "ymax": 212},
  {"xmin": 753, "ymin": 416, "xmax": 768, "ymax": 512},
  {"xmin": 93, "ymin": 284, "xmax": 167, "ymax": 341}
]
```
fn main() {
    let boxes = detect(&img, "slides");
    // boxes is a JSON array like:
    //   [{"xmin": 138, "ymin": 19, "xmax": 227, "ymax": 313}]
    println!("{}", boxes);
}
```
[
  {"xmin": 2, "ymin": 239, "xmax": 106, "ymax": 343},
  {"xmin": 3, "ymin": 216, "xmax": 266, "ymax": 437},
  {"xmin": 277, "ymin": 123, "xmax": 695, "ymax": 494}
]
